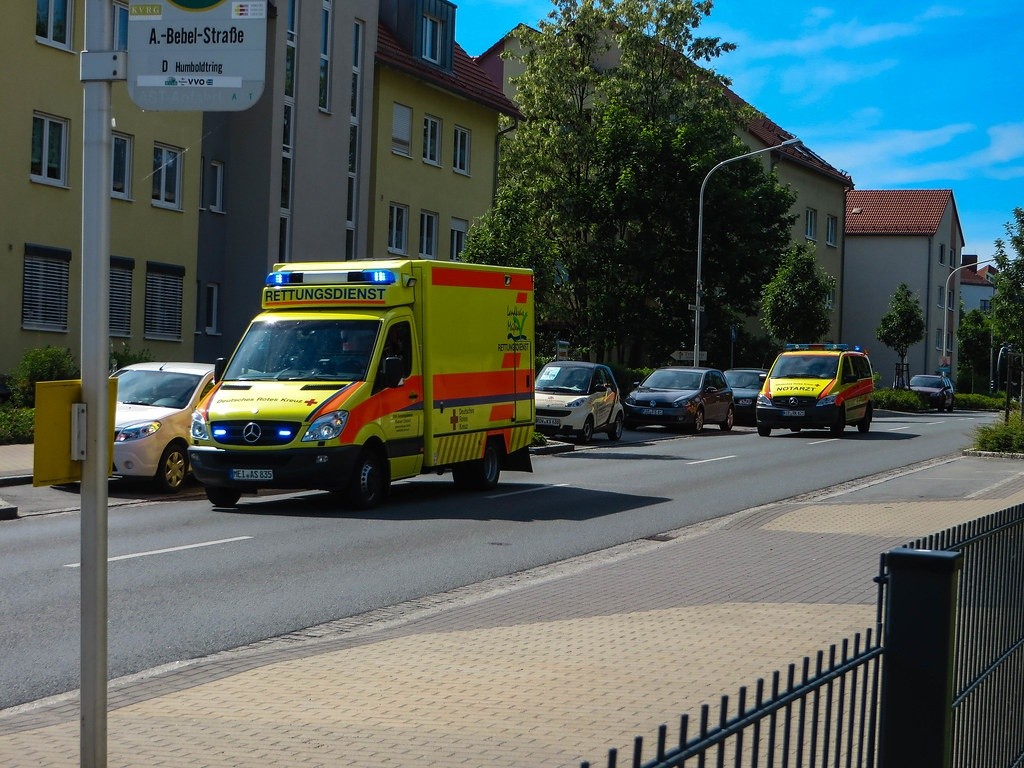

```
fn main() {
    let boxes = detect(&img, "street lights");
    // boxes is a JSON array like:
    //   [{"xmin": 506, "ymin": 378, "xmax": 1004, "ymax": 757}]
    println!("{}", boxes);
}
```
[
  {"xmin": 692, "ymin": 137, "xmax": 802, "ymax": 368},
  {"xmin": 942, "ymin": 256, "xmax": 1000, "ymax": 376}
]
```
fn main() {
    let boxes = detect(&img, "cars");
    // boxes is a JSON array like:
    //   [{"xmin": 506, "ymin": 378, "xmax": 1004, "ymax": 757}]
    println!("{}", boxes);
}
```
[
  {"xmin": 902, "ymin": 374, "xmax": 955, "ymax": 412},
  {"xmin": 535, "ymin": 360, "xmax": 624, "ymax": 444},
  {"xmin": 624, "ymin": 366, "xmax": 736, "ymax": 435},
  {"xmin": 720, "ymin": 366, "xmax": 769, "ymax": 422},
  {"xmin": 109, "ymin": 361, "xmax": 263, "ymax": 493}
]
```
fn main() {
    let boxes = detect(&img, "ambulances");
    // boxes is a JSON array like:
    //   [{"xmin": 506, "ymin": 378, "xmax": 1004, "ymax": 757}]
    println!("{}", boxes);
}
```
[
  {"xmin": 755, "ymin": 343, "xmax": 874, "ymax": 438},
  {"xmin": 187, "ymin": 259, "xmax": 536, "ymax": 510}
]
